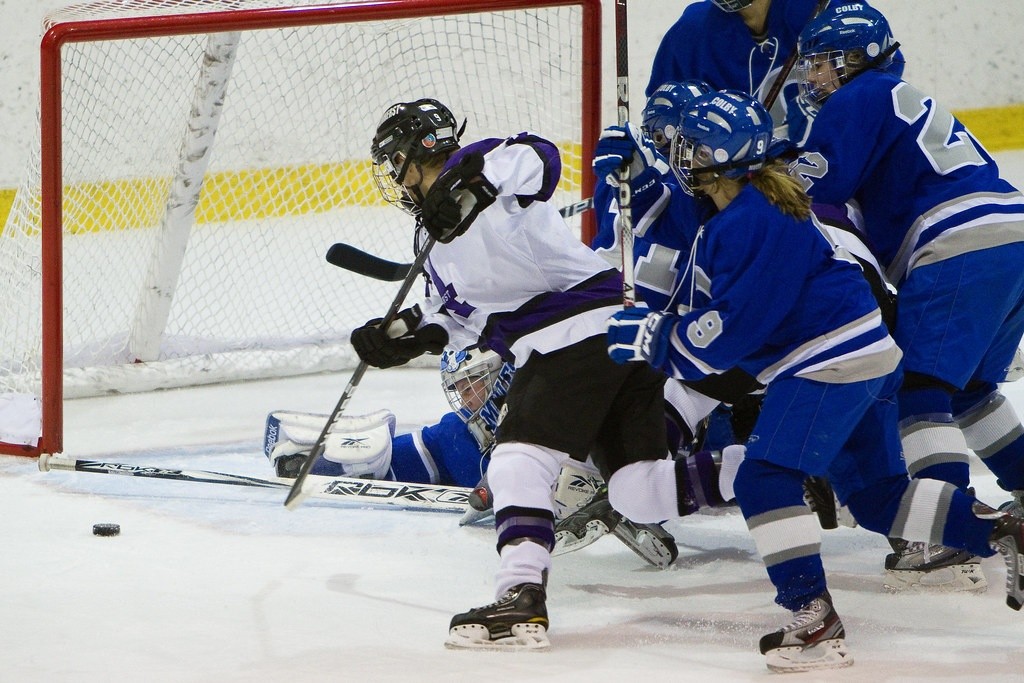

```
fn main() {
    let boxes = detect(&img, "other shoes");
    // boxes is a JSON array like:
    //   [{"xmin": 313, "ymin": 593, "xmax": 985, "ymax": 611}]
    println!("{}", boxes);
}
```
[{"xmin": 274, "ymin": 453, "xmax": 309, "ymax": 479}]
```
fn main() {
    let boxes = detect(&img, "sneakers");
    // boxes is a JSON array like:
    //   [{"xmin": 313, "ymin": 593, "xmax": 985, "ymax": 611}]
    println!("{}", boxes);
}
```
[
  {"xmin": 881, "ymin": 534, "xmax": 989, "ymax": 596},
  {"xmin": 443, "ymin": 567, "xmax": 552, "ymax": 652},
  {"xmin": 758, "ymin": 595, "xmax": 855, "ymax": 675},
  {"xmin": 971, "ymin": 486, "xmax": 1023, "ymax": 612},
  {"xmin": 548, "ymin": 496, "xmax": 621, "ymax": 557},
  {"xmin": 799, "ymin": 472, "xmax": 859, "ymax": 530},
  {"xmin": 591, "ymin": 483, "xmax": 679, "ymax": 571}
]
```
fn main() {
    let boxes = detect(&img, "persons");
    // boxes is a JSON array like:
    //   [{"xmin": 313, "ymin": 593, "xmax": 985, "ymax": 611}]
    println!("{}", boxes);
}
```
[{"xmin": 263, "ymin": 1, "xmax": 1024, "ymax": 673}]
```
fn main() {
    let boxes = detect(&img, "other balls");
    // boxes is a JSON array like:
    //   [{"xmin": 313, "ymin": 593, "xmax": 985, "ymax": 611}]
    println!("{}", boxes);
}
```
[{"xmin": 93, "ymin": 523, "xmax": 121, "ymax": 537}]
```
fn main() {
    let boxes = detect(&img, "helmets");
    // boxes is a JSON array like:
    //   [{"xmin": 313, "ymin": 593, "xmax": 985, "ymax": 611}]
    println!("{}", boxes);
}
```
[
  {"xmin": 668, "ymin": 88, "xmax": 773, "ymax": 199},
  {"xmin": 793, "ymin": 0, "xmax": 906, "ymax": 114},
  {"xmin": 369, "ymin": 98, "xmax": 468, "ymax": 217},
  {"xmin": 439, "ymin": 343, "xmax": 516, "ymax": 453},
  {"xmin": 641, "ymin": 79, "xmax": 716, "ymax": 163}
]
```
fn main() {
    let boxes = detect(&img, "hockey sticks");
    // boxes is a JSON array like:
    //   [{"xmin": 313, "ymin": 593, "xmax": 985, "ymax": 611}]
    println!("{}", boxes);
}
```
[
  {"xmin": 283, "ymin": 232, "xmax": 442, "ymax": 510},
  {"xmin": 38, "ymin": 451, "xmax": 475, "ymax": 512},
  {"xmin": 614, "ymin": 0, "xmax": 636, "ymax": 311},
  {"xmin": 326, "ymin": 197, "xmax": 595, "ymax": 283}
]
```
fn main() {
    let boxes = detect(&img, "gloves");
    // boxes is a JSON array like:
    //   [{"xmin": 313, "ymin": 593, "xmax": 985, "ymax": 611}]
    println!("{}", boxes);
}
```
[
  {"xmin": 350, "ymin": 308, "xmax": 449, "ymax": 370},
  {"xmin": 606, "ymin": 305, "xmax": 681, "ymax": 379},
  {"xmin": 422, "ymin": 149, "xmax": 499, "ymax": 244},
  {"xmin": 592, "ymin": 120, "xmax": 671, "ymax": 234}
]
